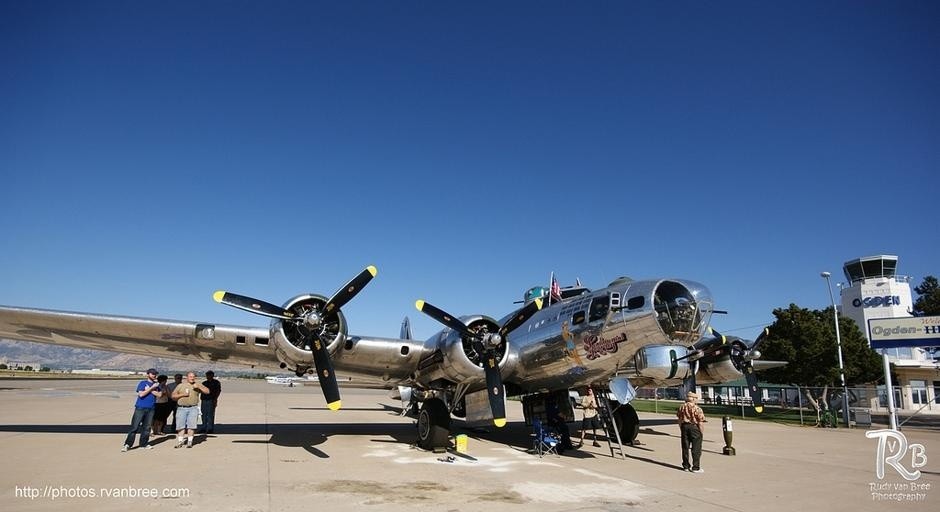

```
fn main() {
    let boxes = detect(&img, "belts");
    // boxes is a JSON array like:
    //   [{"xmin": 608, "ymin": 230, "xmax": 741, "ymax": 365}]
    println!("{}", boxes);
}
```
[{"xmin": 177, "ymin": 404, "xmax": 198, "ymax": 408}]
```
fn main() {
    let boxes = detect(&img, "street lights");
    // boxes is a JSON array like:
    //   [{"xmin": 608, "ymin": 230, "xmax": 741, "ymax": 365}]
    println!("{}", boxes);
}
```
[{"xmin": 820, "ymin": 271, "xmax": 850, "ymax": 426}]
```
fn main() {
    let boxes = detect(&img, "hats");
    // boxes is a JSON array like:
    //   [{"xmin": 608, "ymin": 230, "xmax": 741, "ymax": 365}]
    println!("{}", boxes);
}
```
[
  {"xmin": 686, "ymin": 391, "xmax": 699, "ymax": 399},
  {"xmin": 147, "ymin": 369, "xmax": 159, "ymax": 375}
]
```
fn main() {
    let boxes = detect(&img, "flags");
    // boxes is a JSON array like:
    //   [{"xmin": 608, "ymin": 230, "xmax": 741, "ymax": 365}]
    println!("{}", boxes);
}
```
[{"xmin": 552, "ymin": 273, "xmax": 560, "ymax": 302}]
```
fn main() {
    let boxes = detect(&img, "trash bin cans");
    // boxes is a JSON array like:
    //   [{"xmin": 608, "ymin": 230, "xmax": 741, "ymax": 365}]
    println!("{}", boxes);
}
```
[{"xmin": 855, "ymin": 408, "xmax": 871, "ymax": 428}]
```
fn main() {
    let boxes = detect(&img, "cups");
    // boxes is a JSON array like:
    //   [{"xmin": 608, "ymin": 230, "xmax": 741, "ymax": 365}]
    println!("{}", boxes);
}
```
[{"xmin": 186, "ymin": 389, "xmax": 190, "ymax": 394}]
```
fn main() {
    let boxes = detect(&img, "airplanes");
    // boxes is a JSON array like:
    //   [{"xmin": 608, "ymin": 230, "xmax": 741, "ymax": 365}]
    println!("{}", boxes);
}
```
[{"xmin": 0, "ymin": 266, "xmax": 796, "ymax": 451}]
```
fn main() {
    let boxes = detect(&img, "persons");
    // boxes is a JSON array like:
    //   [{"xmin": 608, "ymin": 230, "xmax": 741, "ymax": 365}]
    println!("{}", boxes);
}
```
[
  {"xmin": 161, "ymin": 374, "xmax": 182, "ymax": 430},
  {"xmin": 121, "ymin": 369, "xmax": 163, "ymax": 452},
  {"xmin": 677, "ymin": 392, "xmax": 705, "ymax": 472},
  {"xmin": 196, "ymin": 371, "xmax": 221, "ymax": 433},
  {"xmin": 579, "ymin": 387, "xmax": 601, "ymax": 447},
  {"xmin": 153, "ymin": 375, "xmax": 171, "ymax": 435},
  {"xmin": 171, "ymin": 372, "xmax": 210, "ymax": 448}
]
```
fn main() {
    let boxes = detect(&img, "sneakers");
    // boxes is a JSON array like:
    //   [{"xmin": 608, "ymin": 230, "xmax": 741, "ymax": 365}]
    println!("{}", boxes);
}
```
[
  {"xmin": 579, "ymin": 441, "xmax": 584, "ymax": 447},
  {"xmin": 140, "ymin": 444, "xmax": 153, "ymax": 450},
  {"xmin": 120, "ymin": 443, "xmax": 130, "ymax": 452},
  {"xmin": 684, "ymin": 468, "xmax": 689, "ymax": 472},
  {"xmin": 187, "ymin": 441, "xmax": 193, "ymax": 448},
  {"xmin": 692, "ymin": 467, "xmax": 704, "ymax": 473},
  {"xmin": 592, "ymin": 441, "xmax": 601, "ymax": 447},
  {"xmin": 174, "ymin": 441, "xmax": 184, "ymax": 448}
]
